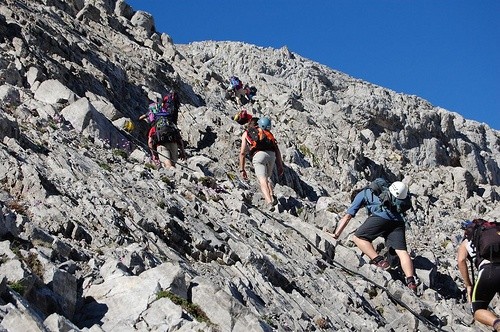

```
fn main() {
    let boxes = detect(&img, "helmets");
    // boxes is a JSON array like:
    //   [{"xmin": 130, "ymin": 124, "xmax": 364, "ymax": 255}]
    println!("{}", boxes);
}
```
[
  {"xmin": 257, "ymin": 118, "xmax": 270, "ymax": 129},
  {"xmin": 389, "ymin": 181, "xmax": 408, "ymax": 199},
  {"xmin": 356, "ymin": 179, "xmax": 371, "ymax": 190}
]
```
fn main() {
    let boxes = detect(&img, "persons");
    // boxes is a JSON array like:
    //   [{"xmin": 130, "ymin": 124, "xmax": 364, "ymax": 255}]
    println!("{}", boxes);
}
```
[
  {"xmin": 325, "ymin": 177, "xmax": 417, "ymax": 292},
  {"xmin": 244, "ymin": 84, "xmax": 253, "ymax": 102},
  {"xmin": 139, "ymin": 91, "xmax": 183, "ymax": 168},
  {"xmin": 239, "ymin": 116, "xmax": 284, "ymax": 212},
  {"xmin": 227, "ymin": 76, "xmax": 246, "ymax": 105},
  {"xmin": 457, "ymin": 218, "xmax": 500, "ymax": 332}
]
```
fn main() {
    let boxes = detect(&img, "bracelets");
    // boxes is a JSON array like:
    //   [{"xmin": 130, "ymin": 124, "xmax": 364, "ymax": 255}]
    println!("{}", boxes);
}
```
[
  {"xmin": 240, "ymin": 169, "xmax": 244, "ymax": 173},
  {"xmin": 335, "ymin": 234, "xmax": 339, "ymax": 237}
]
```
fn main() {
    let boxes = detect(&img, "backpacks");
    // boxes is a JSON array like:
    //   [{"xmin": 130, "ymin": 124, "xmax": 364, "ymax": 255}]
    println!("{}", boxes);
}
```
[
  {"xmin": 156, "ymin": 119, "xmax": 175, "ymax": 142},
  {"xmin": 465, "ymin": 219, "xmax": 500, "ymax": 261},
  {"xmin": 371, "ymin": 178, "xmax": 407, "ymax": 212},
  {"xmin": 244, "ymin": 126, "xmax": 277, "ymax": 152},
  {"xmin": 230, "ymin": 76, "xmax": 240, "ymax": 87}
]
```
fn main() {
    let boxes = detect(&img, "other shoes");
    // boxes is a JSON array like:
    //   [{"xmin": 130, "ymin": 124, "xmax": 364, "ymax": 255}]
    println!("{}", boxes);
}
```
[
  {"xmin": 270, "ymin": 195, "xmax": 278, "ymax": 205},
  {"xmin": 405, "ymin": 276, "xmax": 417, "ymax": 292},
  {"xmin": 266, "ymin": 203, "xmax": 275, "ymax": 211},
  {"xmin": 369, "ymin": 255, "xmax": 391, "ymax": 271}
]
